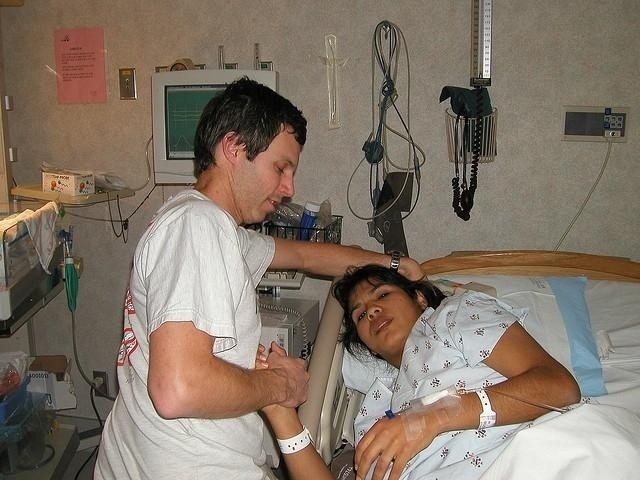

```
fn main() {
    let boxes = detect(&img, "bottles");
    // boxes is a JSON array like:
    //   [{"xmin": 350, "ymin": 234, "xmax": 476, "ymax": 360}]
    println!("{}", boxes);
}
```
[{"xmin": 296, "ymin": 199, "xmax": 320, "ymax": 242}]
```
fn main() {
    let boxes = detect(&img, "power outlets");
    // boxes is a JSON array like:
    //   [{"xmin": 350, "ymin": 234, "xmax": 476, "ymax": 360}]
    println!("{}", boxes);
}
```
[{"xmin": 93, "ymin": 370, "xmax": 109, "ymax": 397}]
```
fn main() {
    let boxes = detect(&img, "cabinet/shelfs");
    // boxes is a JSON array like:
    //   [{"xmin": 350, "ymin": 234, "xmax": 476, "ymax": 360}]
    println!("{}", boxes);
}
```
[{"xmin": 0, "ymin": 183, "xmax": 135, "ymax": 480}]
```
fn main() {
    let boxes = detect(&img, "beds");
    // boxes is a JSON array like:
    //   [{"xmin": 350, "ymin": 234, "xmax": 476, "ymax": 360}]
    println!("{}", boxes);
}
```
[{"xmin": 294, "ymin": 249, "xmax": 640, "ymax": 480}]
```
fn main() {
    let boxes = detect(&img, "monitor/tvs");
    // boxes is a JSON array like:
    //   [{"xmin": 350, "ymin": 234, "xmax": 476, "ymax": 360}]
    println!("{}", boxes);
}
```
[{"xmin": 150, "ymin": 69, "xmax": 280, "ymax": 184}]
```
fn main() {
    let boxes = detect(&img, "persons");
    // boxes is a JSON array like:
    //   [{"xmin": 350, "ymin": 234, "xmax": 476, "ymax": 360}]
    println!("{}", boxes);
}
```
[
  {"xmin": 87, "ymin": 74, "xmax": 431, "ymax": 480},
  {"xmin": 239, "ymin": 257, "xmax": 595, "ymax": 480}
]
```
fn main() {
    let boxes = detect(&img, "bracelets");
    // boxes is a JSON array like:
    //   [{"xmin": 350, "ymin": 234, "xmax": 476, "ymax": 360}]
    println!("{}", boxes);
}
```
[
  {"xmin": 388, "ymin": 248, "xmax": 405, "ymax": 271},
  {"xmin": 276, "ymin": 425, "xmax": 316, "ymax": 455},
  {"xmin": 475, "ymin": 390, "xmax": 497, "ymax": 429}
]
